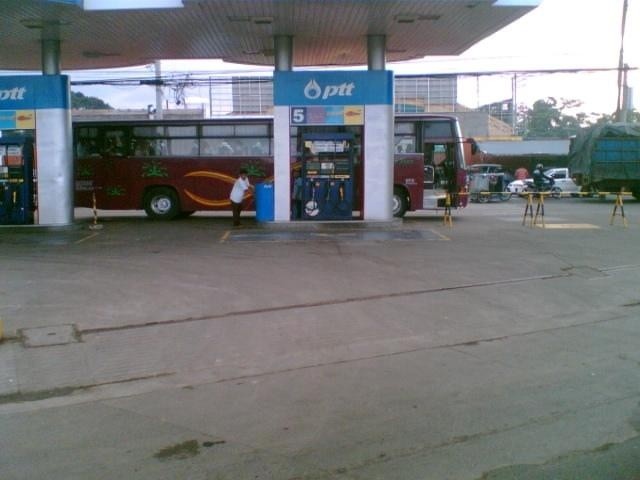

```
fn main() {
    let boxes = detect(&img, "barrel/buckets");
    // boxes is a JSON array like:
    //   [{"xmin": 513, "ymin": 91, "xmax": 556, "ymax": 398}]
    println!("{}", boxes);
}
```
[{"xmin": 254, "ymin": 181, "xmax": 274, "ymax": 222}]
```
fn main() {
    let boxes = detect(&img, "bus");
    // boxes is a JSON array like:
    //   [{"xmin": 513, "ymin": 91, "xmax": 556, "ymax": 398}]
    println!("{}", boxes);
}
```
[{"xmin": 72, "ymin": 115, "xmax": 470, "ymax": 221}]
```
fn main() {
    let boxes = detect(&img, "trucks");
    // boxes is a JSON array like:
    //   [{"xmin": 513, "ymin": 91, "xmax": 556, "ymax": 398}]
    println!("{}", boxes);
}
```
[{"xmin": 567, "ymin": 123, "xmax": 640, "ymax": 199}]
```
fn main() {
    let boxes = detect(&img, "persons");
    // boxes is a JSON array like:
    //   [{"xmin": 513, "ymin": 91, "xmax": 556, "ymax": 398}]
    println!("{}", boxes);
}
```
[
  {"xmin": 396, "ymin": 144, "xmax": 403, "ymax": 154},
  {"xmin": 406, "ymin": 143, "xmax": 415, "ymax": 152},
  {"xmin": 513, "ymin": 162, "xmax": 530, "ymax": 180},
  {"xmin": 532, "ymin": 162, "xmax": 554, "ymax": 200},
  {"xmin": 229, "ymin": 167, "xmax": 255, "ymax": 228}
]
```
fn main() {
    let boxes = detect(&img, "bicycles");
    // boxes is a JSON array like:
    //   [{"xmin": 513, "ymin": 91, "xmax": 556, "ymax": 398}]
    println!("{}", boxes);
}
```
[{"xmin": 478, "ymin": 179, "xmax": 512, "ymax": 203}]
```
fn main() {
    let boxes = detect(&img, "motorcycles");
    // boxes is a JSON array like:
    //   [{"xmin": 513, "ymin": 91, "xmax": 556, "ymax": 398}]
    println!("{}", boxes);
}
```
[{"xmin": 523, "ymin": 176, "xmax": 564, "ymax": 198}]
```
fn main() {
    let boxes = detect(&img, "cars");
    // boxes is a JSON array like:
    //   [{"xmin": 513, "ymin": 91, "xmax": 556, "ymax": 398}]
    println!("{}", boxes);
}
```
[{"xmin": 507, "ymin": 168, "xmax": 593, "ymax": 199}]
